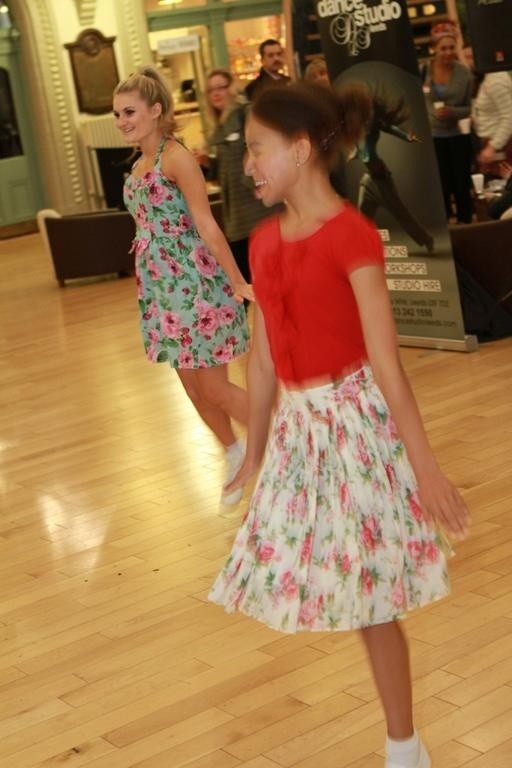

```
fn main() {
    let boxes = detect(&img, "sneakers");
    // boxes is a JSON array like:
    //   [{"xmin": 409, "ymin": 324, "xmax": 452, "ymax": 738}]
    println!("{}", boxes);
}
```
[{"xmin": 222, "ymin": 446, "xmax": 252, "ymax": 507}]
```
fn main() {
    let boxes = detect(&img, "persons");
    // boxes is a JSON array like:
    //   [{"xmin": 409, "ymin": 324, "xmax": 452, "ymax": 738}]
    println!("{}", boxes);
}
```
[
  {"xmin": 113, "ymin": 64, "xmax": 256, "ymax": 506},
  {"xmin": 220, "ymin": 86, "xmax": 473, "ymax": 768}
]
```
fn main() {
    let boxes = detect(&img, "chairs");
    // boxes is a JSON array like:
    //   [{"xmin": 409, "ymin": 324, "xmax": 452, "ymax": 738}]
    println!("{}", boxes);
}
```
[{"xmin": 45, "ymin": 208, "xmax": 135, "ymax": 286}]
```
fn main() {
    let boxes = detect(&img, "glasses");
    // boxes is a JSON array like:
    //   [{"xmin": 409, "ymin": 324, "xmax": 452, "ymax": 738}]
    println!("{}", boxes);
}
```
[{"xmin": 207, "ymin": 85, "xmax": 228, "ymax": 93}]
